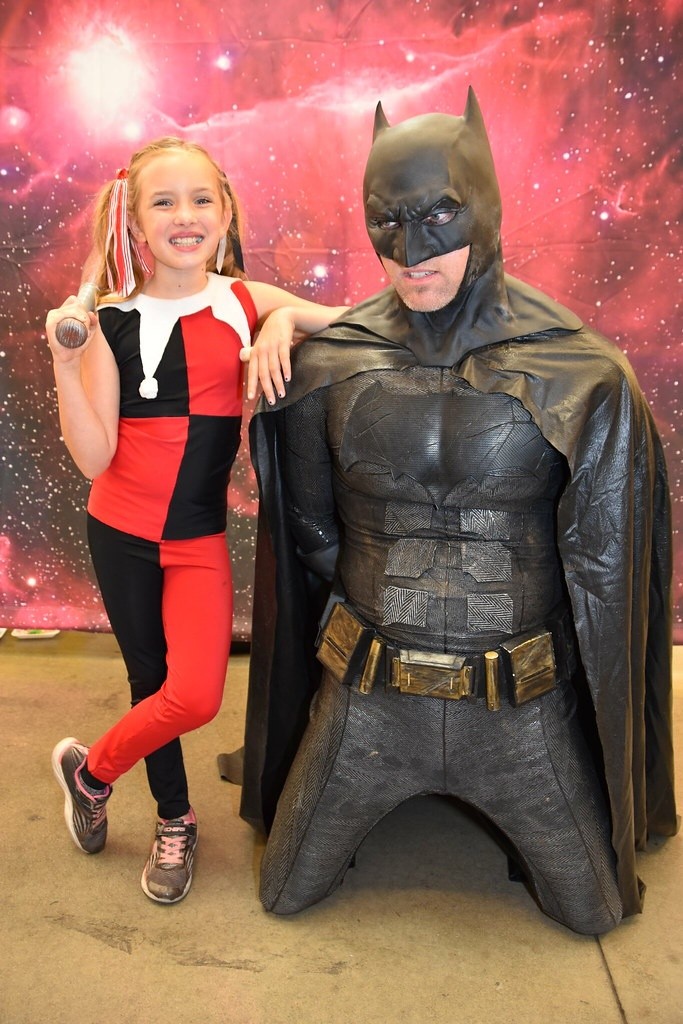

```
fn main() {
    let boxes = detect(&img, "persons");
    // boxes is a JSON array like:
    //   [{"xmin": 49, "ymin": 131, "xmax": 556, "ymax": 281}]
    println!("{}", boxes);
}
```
[
  {"xmin": 218, "ymin": 85, "xmax": 679, "ymax": 935},
  {"xmin": 45, "ymin": 136, "xmax": 352, "ymax": 905}
]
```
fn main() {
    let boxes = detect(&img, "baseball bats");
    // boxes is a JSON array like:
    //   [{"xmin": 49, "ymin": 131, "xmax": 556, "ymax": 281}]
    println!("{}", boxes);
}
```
[{"xmin": 55, "ymin": 244, "xmax": 113, "ymax": 349}]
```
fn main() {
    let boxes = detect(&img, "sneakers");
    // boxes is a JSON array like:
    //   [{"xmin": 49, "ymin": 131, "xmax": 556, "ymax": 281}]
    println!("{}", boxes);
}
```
[
  {"xmin": 53, "ymin": 736, "xmax": 111, "ymax": 855},
  {"xmin": 140, "ymin": 804, "xmax": 198, "ymax": 903}
]
